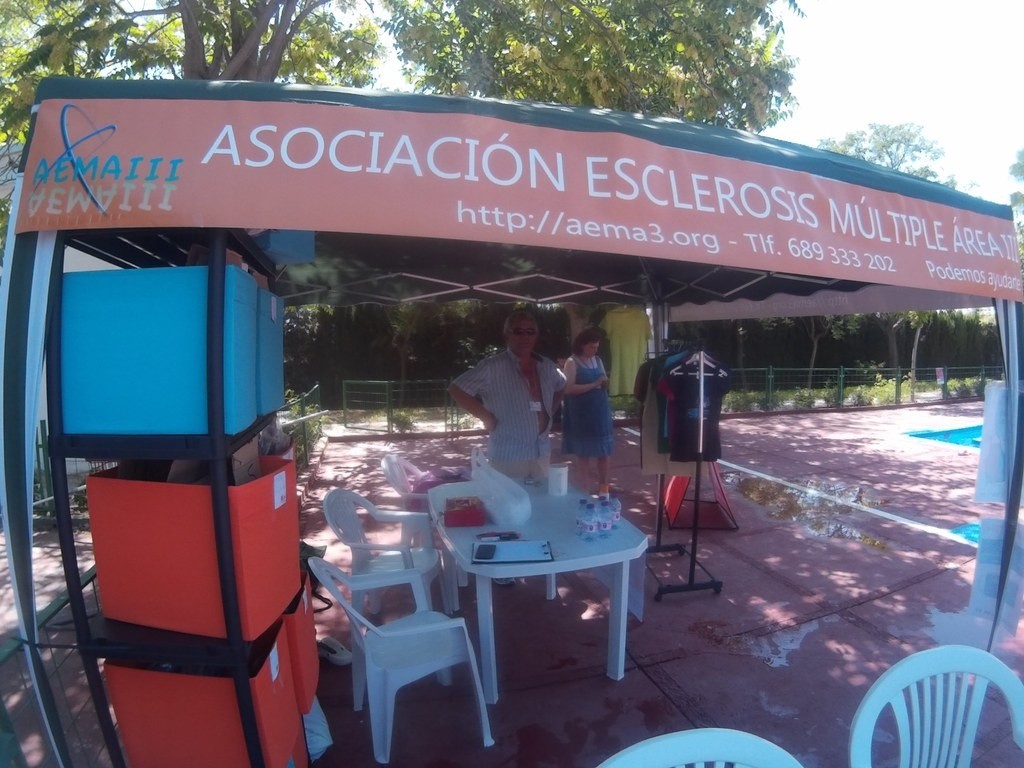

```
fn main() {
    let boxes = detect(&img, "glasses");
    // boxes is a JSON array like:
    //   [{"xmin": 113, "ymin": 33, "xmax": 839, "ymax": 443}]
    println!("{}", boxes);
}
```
[{"xmin": 506, "ymin": 326, "xmax": 539, "ymax": 336}]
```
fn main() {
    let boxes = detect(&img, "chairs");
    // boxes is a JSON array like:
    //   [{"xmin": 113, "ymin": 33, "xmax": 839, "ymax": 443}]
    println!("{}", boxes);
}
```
[
  {"xmin": 308, "ymin": 453, "xmax": 495, "ymax": 763},
  {"xmin": 595, "ymin": 728, "xmax": 805, "ymax": 768},
  {"xmin": 847, "ymin": 644, "xmax": 1023, "ymax": 768}
]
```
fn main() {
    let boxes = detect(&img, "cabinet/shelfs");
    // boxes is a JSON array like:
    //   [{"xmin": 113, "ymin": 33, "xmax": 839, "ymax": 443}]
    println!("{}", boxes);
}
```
[{"xmin": 46, "ymin": 227, "xmax": 279, "ymax": 768}]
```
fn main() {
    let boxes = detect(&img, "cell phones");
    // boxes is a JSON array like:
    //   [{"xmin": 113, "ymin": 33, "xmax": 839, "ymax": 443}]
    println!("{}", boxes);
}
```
[{"xmin": 475, "ymin": 544, "xmax": 496, "ymax": 559}]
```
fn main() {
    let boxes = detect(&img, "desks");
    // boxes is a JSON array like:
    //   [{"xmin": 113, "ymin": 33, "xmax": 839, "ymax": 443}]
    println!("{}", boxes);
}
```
[{"xmin": 427, "ymin": 476, "xmax": 649, "ymax": 705}]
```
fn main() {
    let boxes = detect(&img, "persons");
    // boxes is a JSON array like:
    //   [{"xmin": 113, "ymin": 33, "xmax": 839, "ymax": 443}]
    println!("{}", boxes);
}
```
[
  {"xmin": 564, "ymin": 329, "xmax": 613, "ymax": 500},
  {"xmin": 450, "ymin": 310, "xmax": 568, "ymax": 585}
]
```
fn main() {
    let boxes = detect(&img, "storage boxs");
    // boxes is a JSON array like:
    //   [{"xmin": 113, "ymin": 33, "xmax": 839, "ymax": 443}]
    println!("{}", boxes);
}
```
[
  {"xmin": 444, "ymin": 497, "xmax": 484, "ymax": 527},
  {"xmin": 60, "ymin": 266, "xmax": 285, "ymax": 435},
  {"xmin": 85, "ymin": 437, "xmax": 319, "ymax": 767}
]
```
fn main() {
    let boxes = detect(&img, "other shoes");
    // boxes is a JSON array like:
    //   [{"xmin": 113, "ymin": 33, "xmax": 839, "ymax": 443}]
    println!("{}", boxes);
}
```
[{"xmin": 321, "ymin": 637, "xmax": 353, "ymax": 665}]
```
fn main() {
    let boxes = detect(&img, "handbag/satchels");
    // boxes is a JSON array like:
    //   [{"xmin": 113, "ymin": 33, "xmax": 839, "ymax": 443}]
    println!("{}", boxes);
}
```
[{"xmin": 470, "ymin": 447, "xmax": 531, "ymax": 531}]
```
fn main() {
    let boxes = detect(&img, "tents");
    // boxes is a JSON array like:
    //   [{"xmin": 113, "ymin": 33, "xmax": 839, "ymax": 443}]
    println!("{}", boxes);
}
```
[{"xmin": 3, "ymin": 76, "xmax": 1023, "ymax": 768}]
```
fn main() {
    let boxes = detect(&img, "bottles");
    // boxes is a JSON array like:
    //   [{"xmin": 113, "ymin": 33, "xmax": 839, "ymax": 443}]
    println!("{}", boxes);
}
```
[
  {"xmin": 598, "ymin": 485, "xmax": 609, "ymax": 502},
  {"xmin": 597, "ymin": 496, "xmax": 612, "ymax": 539},
  {"xmin": 581, "ymin": 504, "xmax": 596, "ymax": 544},
  {"xmin": 574, "ymin": 499, "xmax": 587, "ymax": 535},
  {"xmin": 610, "ymin": 495, "xmax": 622, "ymax": 529}
]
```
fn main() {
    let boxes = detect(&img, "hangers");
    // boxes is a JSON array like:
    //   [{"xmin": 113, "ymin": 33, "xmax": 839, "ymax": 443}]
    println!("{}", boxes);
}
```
[{"xmin": 643, "ymin": 337, "xmax": 729, "ymax": 378}]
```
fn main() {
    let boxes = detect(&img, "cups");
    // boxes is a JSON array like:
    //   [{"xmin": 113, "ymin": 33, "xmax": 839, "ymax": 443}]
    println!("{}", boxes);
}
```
[{"xmin": 548, "ymin": 463, "xmax": 569, "ymax": 498}]
